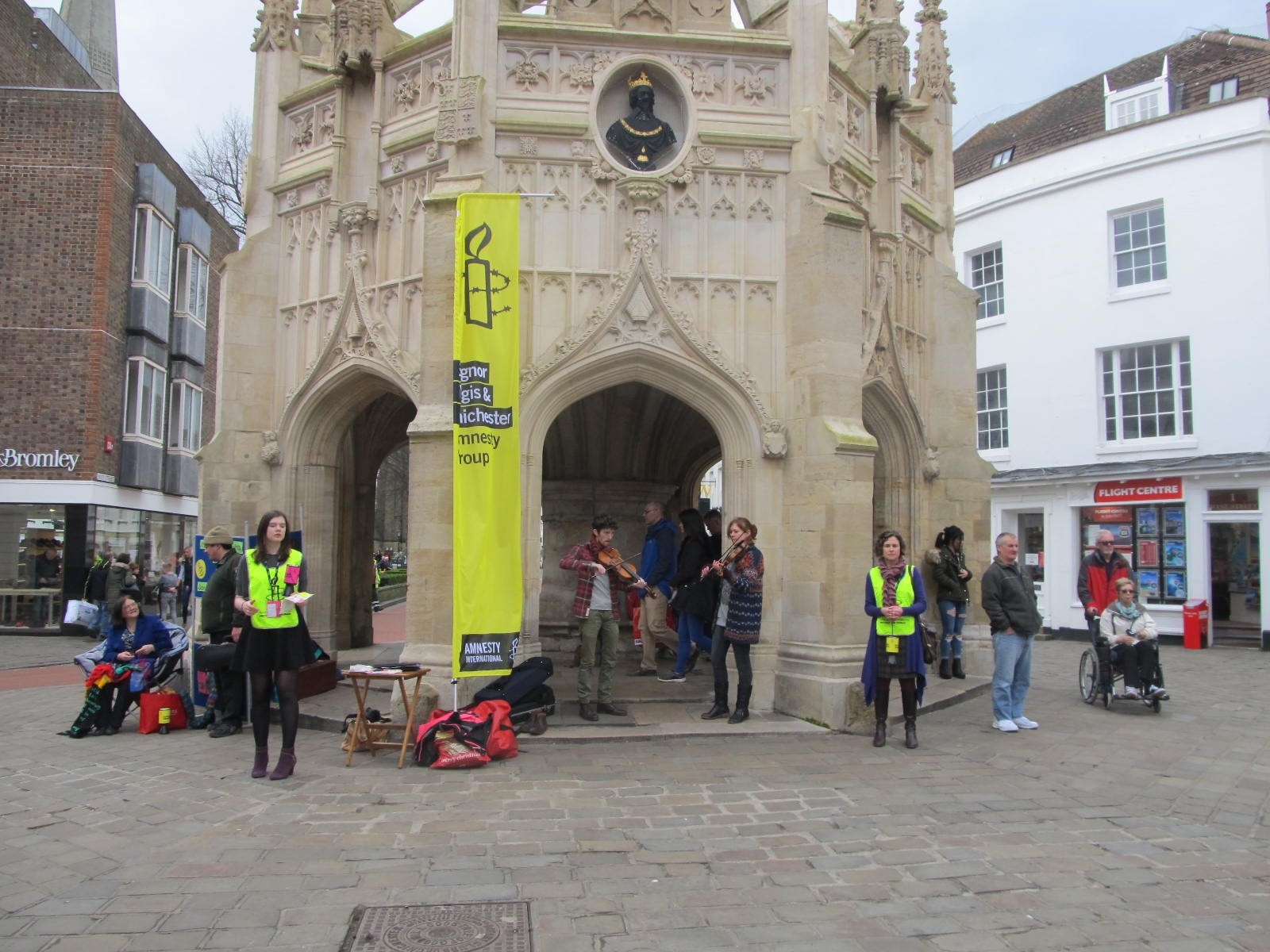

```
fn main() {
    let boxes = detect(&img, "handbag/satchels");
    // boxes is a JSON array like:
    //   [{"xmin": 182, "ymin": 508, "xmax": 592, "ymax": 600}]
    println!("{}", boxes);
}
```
[
  {"xmin": 920, "ymin": 627, "xmax": 937, "ymax": 665},
  {"xmin": 64, "ymin": 599, "xmax": 98, "ymax": 626},
  {"xmin": 414, "ymin": 700, "xmax": 518, "ymax": 765},
  {"xmin": 633, "ymin": 606, "xmax": 675, "ymax": 647},
  {"xmin": 138, "ymin": 692, "xmax": 187, "ymax": 735},
  {"xmin": 120, "ymin": 584, "xmax": 143, "ymax": 603},
  {"xmin": 340, "ymin": 716, "xmax": 388, "ymax": 751}
]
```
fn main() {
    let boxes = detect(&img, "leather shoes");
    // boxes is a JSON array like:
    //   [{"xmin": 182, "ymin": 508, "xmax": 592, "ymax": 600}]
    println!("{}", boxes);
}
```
[
  {"xmin": 597, "ymin": 702, "xmax": 628, "ymax": 716},
  {"xmin": 579, "ymin": 704, "xmax": 600, "ymax": 721}
]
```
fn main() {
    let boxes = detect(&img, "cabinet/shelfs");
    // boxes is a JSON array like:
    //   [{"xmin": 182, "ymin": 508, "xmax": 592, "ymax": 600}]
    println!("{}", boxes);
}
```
[{"xmin": 17, "ymin": 528, "xmax": 64, "ymax": 583}]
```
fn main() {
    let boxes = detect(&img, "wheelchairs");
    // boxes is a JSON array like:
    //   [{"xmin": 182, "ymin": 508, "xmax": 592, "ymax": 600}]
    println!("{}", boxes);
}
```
[{"xmin": 1077, "ymin": 612, "xmax": 1169, "ymax": 715}]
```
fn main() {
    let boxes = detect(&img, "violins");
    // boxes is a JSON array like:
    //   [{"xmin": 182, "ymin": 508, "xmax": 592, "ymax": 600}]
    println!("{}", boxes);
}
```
[
  {"xmin": 715, "ymin": 539, "xmax": 748, "ymax": 578},
  {"xmin": 597, "ymin": 545, "xmax": 659, "ymax": 600}
]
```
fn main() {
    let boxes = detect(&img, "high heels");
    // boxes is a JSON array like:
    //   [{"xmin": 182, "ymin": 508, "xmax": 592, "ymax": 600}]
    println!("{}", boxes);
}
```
[
  {"xmin": 252, "ymin": 747, "xmax": 269, "ymax": 779},
  {"xmin": 269, "ymin": 747, "xmax": 297, "ymax": 779}
]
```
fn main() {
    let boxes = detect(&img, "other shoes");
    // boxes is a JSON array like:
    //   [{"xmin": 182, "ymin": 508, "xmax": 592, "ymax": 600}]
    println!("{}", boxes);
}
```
[
  {"xmin": 192, "ymin": 711, "xmax": 214, "ymax": 730},
  {"xmin": 628, "ymin": 668, "xmax": 657, "ymax": 676},
  {"xmin": 209, "ymin": 722, "xmax": 243, "ymax": 738},
  {"xmin": 658, "ymin": 671, "xmax": 686, "ymax": 682},
  {"xmin": 103, "ymin": 725, "xmax": 118, "ymax": 735},
  {"xmin": 99, "ymin": 634, "xmax": 106, "ymax": 641},
  {"xmin": 685, "ymin": 650, "xmax": 699, "ymax": 673},
  {"xmin": 93, "ymin": 724, "xmax": 105, "ymax": 736},
  {"xmin": 87, "ymin": 628, "xmax": 97, "ymax": 638}
]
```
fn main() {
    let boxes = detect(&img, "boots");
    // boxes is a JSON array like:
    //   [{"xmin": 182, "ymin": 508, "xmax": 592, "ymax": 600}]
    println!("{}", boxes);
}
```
[
  {"xmin": 939, "ymin": 659, "xmax": 952, "ymax": 679},
  {"xmin": 953, "ymin": 658, "xmax": 965, "ymax": 679},
  {"xmin": 728, "ymin": 684, "xmax": 753, "ymax": 724},
  {"xmin": 874, "ymin": 692, "xmax": 889, "ymax": 747},
  {"xmin": 901, "ymin": 686, "xmax": 918, "ymax": 749},
  {"xmin": 701, "ymin": 681, "xmax": 730, "ymax": 720}
]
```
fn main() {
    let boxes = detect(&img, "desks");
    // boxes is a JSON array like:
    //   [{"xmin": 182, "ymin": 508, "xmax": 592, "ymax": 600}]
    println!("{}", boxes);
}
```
[
  {"xmin": 0, "ymin": 588, "xmax": 62, "ymax": 627},
  {"xmin": 342, "ymin": 668, "xmax": 431, "ymax": 768}
]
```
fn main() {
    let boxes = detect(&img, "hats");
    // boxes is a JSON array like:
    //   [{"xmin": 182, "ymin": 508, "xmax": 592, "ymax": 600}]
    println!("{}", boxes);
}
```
[{"xmin": 203, "ymin": 525, "xmax": 234, "ymax": 545}]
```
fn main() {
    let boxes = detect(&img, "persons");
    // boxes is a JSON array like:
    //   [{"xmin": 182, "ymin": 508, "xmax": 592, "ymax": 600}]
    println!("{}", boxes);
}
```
[
  {"xmin": 702, "ymin": 518, "xmax": 764, "ymax": 725},
  {"xmin": 861, "ymin": 530, "xmax": 927, "ymax": 749},
  {"xmin": 199, "ymin": 526, "xmax": 249, "ymax": 736},
  {"xmin": 27, "ymin": 532, "xmax": 60, "ymax": 628},
  {"xmin": 627, "ymin": 497, "xmax": 681, "ymax": 678},
  {"xmin": 1077, "ymin": 530, "xmax": 1139, "ymax": 701},
  {"xmin": 234, "ymin": 510, "xmax": 308, "ymax": 780},
  {"xmin": 1099, "ymin": 577, "xmax": 1167, "ymax": 701},
  {"xmin": 92, "ymin": 595, "xmax": 173, "ymax": 736},
  {"xmin": 558, "ymin": 512, "xmax": 647, "ymax": 722},
  {"xmin": 657, "ymin": 507, "xmax": 712, "ymax": 683},
  {"xmin": 606, "ymin": 71, "xmax": 677, "ymax": 172},
  {"xmin": 695, "ymin": 509, "xmax": 722, "ymax": 660},
  {"xmin": 83, "ymin": 545, "xmax": 194, "ymax": 641},
  {"xmin": 925, "ymin": 526, "xmax": 973, "ymax": 679},
  {"xmin": 981, "ymin": 532, "xmax": 1043, "ymax": 732},
  {"xmin": 372, "ymin": 548, "xmax": 407, "ymax": 613}
]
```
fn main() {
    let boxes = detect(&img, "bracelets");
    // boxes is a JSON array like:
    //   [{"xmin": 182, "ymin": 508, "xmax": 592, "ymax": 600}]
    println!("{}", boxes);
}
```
[{"xmin": 241, "ymin": 603, "xmax": 245, "ymax": 614}]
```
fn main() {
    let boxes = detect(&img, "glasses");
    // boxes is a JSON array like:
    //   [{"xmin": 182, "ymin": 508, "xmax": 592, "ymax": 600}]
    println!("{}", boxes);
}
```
[
  {"xmin": 1122, "ymin": 590, "xmax": 1134, "ymax": 593},
  {"xmin": 1099, "ymin": 541, "xmax": 1116, "ymax": 546}
]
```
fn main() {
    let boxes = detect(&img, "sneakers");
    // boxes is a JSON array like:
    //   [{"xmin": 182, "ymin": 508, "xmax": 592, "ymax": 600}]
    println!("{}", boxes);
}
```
[
  {"xmin": 1145, "ymin": 685, "xmax": 1165, "ymax": 698},
  {"xmin": 1013, "ymin": 717, "xmax": 1038, "ymax": 729},
  {"xmin": 1124, "ymin": 686, "xmax": 1138, "ymax": 698},
  {"xmin": 991, "ymin": 719, "xmax": 1019, "ymax": 732}
]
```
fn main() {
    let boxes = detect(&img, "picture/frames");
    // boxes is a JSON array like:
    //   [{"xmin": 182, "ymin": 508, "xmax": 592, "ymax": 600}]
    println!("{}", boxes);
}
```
[{"xmin": 1133, "ymin": 506, "xmax": 1188, "ymax": 600}]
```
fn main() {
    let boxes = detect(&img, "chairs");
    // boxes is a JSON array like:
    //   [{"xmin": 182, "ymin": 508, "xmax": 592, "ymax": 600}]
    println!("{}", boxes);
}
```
[{"xmin": 73, "ymin": 621, "xmax": 189, "ymax": 722}]
